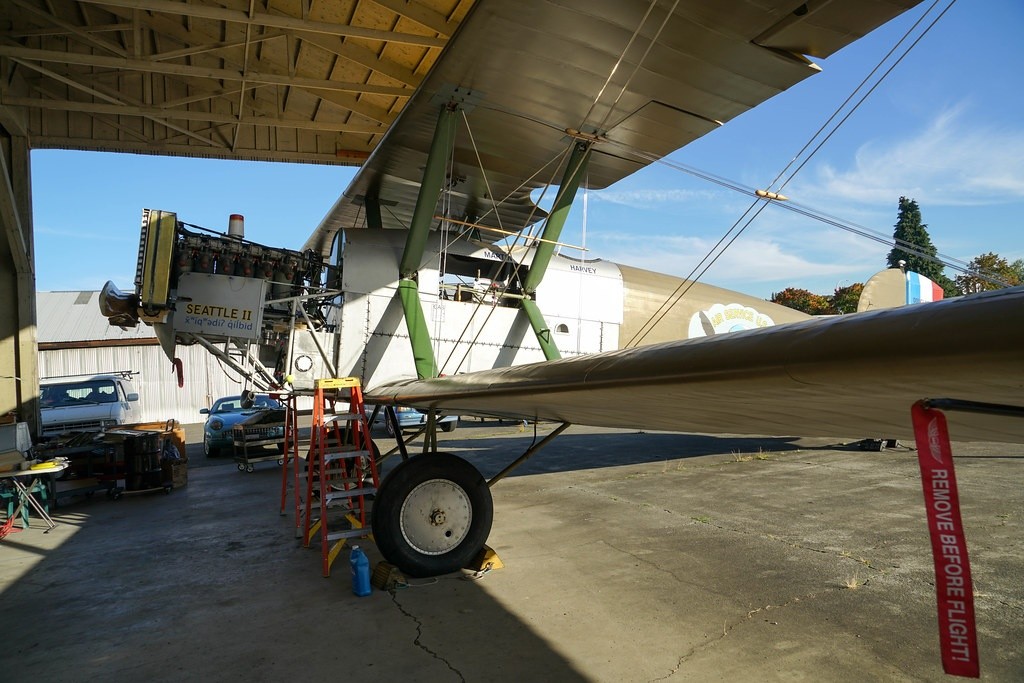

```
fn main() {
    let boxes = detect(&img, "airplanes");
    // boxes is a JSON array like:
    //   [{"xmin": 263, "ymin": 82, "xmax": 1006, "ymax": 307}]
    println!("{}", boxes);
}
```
[{"xmin": 99, "ymin": 0, "xmax": 1024, "ymax": 578}]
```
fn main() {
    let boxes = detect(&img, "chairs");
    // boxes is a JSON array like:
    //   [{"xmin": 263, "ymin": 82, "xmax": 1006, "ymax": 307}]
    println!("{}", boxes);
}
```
[{"xmin": 222, "ymin": 403, "xmax": 234, "ymax": 410}]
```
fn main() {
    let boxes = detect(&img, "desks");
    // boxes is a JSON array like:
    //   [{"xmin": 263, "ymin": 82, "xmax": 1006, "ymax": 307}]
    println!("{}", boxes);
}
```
[{"xmin": 0, "ymin": 462, "xmax": 69, "ymax": 540}]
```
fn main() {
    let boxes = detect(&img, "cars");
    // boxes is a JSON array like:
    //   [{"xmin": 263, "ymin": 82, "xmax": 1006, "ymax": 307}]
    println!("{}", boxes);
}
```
[
  {"xmin": 199, "ymin": 392, "xmax": 294, "ymax": 456},
  {"xmin": 362, "ymin": 401, "xmax": 460, "ymax": 437}
]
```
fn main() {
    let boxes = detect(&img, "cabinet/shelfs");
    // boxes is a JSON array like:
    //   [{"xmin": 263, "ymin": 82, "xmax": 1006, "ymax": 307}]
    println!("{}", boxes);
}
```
[
  {"xmin": 36, "ymin": 440, "xmax": 113, "ymax": 513},
  {"xmin": 232, "ymin": 407, "xmax": 295, "ymax": 464}
]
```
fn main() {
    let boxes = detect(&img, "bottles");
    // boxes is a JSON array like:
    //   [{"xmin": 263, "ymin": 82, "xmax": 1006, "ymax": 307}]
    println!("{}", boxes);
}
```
[{"xmin": 349, "ymin": 545, "xmax": 371, "ymax": 596}]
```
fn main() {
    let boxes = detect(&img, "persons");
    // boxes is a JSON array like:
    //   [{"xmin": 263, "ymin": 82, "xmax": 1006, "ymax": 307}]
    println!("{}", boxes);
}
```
[{"xmin": 260, "ymin": 399, "xmax": 268, "ymax": 407}]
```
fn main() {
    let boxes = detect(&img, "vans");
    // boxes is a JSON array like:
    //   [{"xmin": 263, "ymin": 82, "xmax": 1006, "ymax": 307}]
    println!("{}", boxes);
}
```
[{"xmin": 40, "ymin": 376, "xmax": 141, "ymax": 442}]
